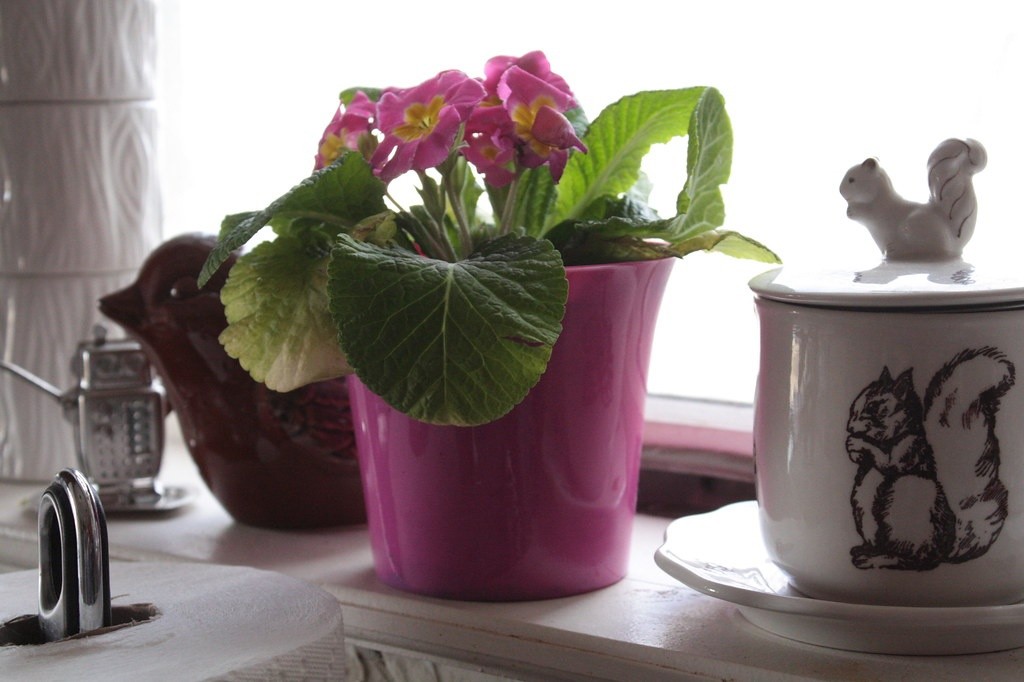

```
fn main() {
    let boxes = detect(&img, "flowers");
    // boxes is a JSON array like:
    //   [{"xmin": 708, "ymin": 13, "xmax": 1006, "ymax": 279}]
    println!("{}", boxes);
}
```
[{"xmin": 194, "ymin": 50, "xmax": 782, "ymax": 428}]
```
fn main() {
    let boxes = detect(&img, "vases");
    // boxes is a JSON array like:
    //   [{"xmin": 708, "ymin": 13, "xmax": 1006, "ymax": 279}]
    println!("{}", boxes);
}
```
[{"xmin": 342, "ymin": 248, "xmax": 677, "ymax": 602}]
